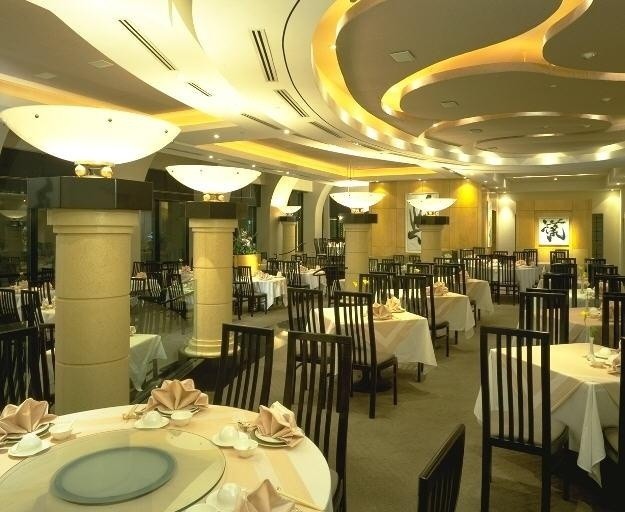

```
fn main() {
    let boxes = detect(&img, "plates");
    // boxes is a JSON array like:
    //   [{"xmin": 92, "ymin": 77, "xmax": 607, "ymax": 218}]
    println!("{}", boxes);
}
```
[
  {"xmin": 212, "ymin": 432, "xmax": 248, "ymax": 448},
  {"xmin": 232, "ymin": 425, "xmax": 304, "ymax": 446},
  {"xmin": 134, "ymin": 404, "xmax": 200, "ymax": 429},
  {"xmin": 206, "ymin": 487, "xmax": 249, "ymax": 511},
  {"xmin": 185, "ymin": 503, "xmax": 220, "ymax": 512},
  {"xmin": 594, "ymin": 352, "xmax": 621, "ymax": 367},
  {"xmin": 0, "ymin": 422, "xmax": 55, "ymax": 456}
]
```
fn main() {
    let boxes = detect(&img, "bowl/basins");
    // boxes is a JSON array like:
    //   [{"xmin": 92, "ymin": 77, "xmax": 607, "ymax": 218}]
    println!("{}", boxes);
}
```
[
  {"xmin": 49, "ymin": 424, "xmax": 72, "ymax": 440},
  {"xmin": 591, "ymin": 357, "xmax": 605, "ymax": 367},
  {"xmin": 232, "ymin": 438, "xmax": 258, "ymax": 458}
]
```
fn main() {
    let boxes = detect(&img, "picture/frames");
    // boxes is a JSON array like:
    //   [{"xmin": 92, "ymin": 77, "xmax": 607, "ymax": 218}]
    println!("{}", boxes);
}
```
[{"xmin": 405, "ymin": 191, "xmax": 441, "ymax": 254}]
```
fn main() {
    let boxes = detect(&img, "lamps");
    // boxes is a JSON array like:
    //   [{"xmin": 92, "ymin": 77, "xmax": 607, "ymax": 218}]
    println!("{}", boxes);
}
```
[
  {"xmin": 405, "ymin": 198, "xmax": 456, "ymax": 216},
  {"xmin": 164, "ymin": 163, "xmax": 261, "ymax": 202},
  {"xmin": 278, "ymin": 205, "xmax": 301, "ymax": 217},
  {"xmin": 0, "ymin": 107, "xmax": 181, "ymax": 179},
  {"xmin": 328, "ymin": 192, "xmax": 386, "ymax": 214}
]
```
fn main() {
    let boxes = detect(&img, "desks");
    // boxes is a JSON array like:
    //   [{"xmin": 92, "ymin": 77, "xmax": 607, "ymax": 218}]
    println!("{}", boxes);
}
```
[
  {"xmin": 0, "ymin": 402, "xmax": 333, "ymax": 512},
  {"xmin": 474, "ymin": 343, "xmax": 621, "ymax": 488},
  {"xmin": 304, "ymin": 304, "xmax": 437, "ymax": 382},
  {"xmin": 40, "ymin": 333, "xmax": 167, "ymax": 404}
]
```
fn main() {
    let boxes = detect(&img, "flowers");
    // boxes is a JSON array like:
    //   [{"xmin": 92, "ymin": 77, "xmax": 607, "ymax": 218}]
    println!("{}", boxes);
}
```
[{"xmin": 233, "ymin": 227, "xmax": 260, "ymax": 254}]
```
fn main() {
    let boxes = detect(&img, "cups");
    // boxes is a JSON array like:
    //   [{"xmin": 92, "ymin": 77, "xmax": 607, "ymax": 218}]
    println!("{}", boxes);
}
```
[
  {"xmin": 600, "ymin": 347, "xmax": 611, "ymax": 356},
  {"xmin": 216, "ymin": 483, "xmax": 246, "ymax": 510},
  {"xmin": 217, "ymin": 423, "xmax": 239, "ymax": 443}
]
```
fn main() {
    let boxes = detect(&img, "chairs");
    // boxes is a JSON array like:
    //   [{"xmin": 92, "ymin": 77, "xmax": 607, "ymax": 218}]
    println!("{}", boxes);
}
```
[
  {"xmin": 232, "ymin": 237, "xmax": 348, "ymax": 319},
  {"xmin": 287, "ymin": 287, "xmax": 356, "ymax": 409},
  {"xmin": 0, "ymin": 255, "xmax": 56, "ymax": 411},
  {"xmin": 519, "ymin": 248, "xmax": 624, "ymax": 348},
  {"xmin": 600, "ymin": 337, "xmax": 624, "ymax": 511},
  {"xmin": 404, "ymin": 272, "xmax": 450, "ymax": 357},
  {"xmin": 212, "ymin": 323, "xmax": 275, "ymax": 414},
  {"xmin": 449, "ymin": 244, "xmax": 542, "ymax": 306},
  {"xmin": 282, "ymin": 331, "xmax": 352, "ymax": 510},
  {"xmin": 129, "ymin": 257, "xmax": 195, "ymax": 320},
  {"xmin": 357, "ymin": 243, "xmax": 494, "ymax": 345},
  {"xmin": 478, "ymin": 325, "xmax": 570, "ymax": 511},
  {"xmin": 332, "ymin": 288, "xmax": 397, "ymax": 418},
  {"xmin": 417, "ymin": 422, "xmax": 465, "ymax": 510}
]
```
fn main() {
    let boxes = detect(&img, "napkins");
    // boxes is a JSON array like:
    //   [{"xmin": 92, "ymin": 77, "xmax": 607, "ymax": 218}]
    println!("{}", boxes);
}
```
[
  {"xmin": 386, "ymin": 297, "xmax": 402, "ymax": 313},
  {"xmin": 372, "ymin": 301, "xmax": 393, "ymax": 321},
  {"xmin": 253, "ymin": 401, "xmax": 306, "ymax": 448},
  {"xmin": 147, "ymin": 379, "xmax": 208, "ymax": 414},
  {"xmin": 605, "ymin": 354, "xmax": 621, "ymax": 375},
  {"xmin": 1, "ymin": 398, "xmax": 57, "ymax": 444},
  {"xmin": 241, "ymin": 478, "xmax": 296, "ymax": 511}
]
